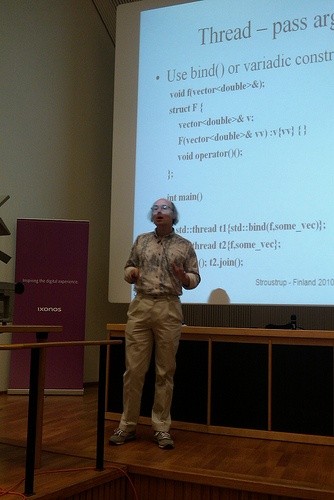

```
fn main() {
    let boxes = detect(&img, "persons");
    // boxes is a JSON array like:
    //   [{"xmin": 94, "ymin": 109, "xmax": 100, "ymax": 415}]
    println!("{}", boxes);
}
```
[{"xmin": 108, "ymin": 198, "xmax": 201, "ymax": 450}]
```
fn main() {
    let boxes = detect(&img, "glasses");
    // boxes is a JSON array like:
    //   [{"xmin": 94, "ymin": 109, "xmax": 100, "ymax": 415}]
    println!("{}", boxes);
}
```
[{"xmin": 151, "ymin": 206, "xmax": 174, "ymax": 212}]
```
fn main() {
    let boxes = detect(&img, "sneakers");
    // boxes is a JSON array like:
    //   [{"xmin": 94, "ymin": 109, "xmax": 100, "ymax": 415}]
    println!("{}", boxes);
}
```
[
  {"xmin": 109, "ymin": 427, "xmax": 137, "ymax": 445},
  {"xmin": 154, "ymin": 431, "xmax": 175, "ymax": 449}
]
